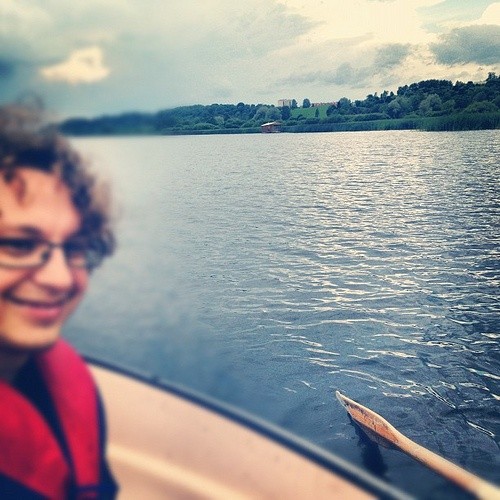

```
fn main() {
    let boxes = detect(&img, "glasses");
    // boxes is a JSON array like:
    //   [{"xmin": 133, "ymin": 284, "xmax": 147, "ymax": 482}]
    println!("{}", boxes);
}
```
[{"xmin": 0, "ymin": 230, "xmax": 108, "ymax": 273}]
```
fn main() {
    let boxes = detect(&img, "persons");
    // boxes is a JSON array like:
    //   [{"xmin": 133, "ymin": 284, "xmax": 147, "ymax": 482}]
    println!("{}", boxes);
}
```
[{"xmin": 1, "ymin": 90, "xmax": 120, "ymax": 500}]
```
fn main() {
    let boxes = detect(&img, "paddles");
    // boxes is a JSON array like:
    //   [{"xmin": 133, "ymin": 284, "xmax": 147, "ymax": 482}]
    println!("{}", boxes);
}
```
[{"xmin": 334, "ymin": 389, "xmax": 500, "ymax": 500}]
